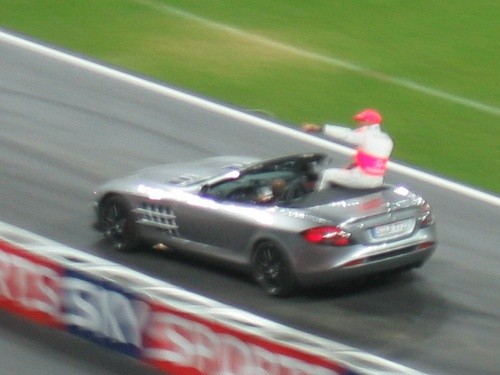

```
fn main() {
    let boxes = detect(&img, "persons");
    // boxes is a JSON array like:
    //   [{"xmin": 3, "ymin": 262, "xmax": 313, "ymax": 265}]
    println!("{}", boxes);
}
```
[
  {"xmin": 304, "ymin": 109, "xmax": 394, "ymax": 192},
  {"xmin": 280, "ymin": 158, "xmax": 318, "ymax": 202}
]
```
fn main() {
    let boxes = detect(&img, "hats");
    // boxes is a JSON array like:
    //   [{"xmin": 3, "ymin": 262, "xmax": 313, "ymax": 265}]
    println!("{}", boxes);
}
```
[{"xmin": 354, "ymin": 109, "xmax": 381, "ymax": 123}]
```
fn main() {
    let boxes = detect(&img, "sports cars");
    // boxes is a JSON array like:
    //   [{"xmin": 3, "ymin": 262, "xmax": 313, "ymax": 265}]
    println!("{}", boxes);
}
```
[{"xmin": 91, "ymin": 153, "xmax": 440, "ymax": 298}]
